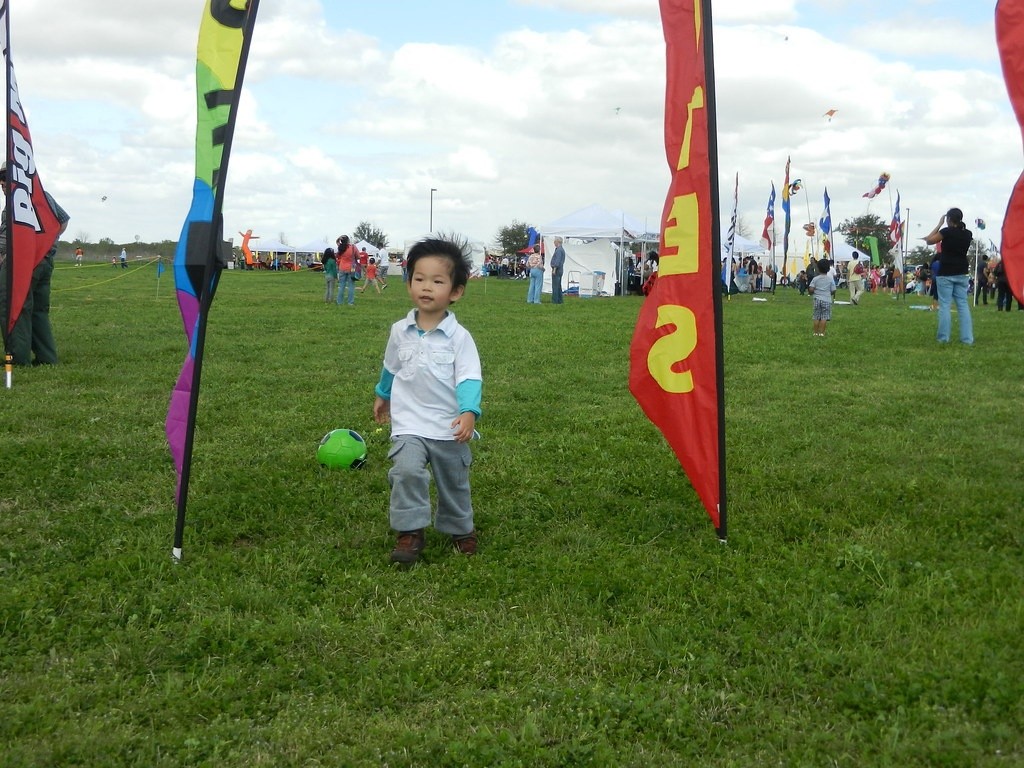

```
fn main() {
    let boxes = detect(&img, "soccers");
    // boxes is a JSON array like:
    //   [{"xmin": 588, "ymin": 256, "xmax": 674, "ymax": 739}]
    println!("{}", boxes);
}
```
[{"xmin": 316, "ymin": 428, "xmax": 368, "ymax": 471}]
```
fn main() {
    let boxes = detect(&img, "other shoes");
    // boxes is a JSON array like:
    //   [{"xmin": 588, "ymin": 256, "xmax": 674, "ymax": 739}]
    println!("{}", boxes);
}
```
[
  {"xmin": 382, "ymin": 284, "xmax": 387, "ymax": 290},
  {"xmin": 75, "ymin": 264, "xmax": 82, "ymax": 266},
  {"xmin": 812, "ymin": 333, "xmax": 826, "ymax": 337}
]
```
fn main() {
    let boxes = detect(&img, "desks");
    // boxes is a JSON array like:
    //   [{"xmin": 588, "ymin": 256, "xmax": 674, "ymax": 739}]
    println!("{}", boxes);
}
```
[{"xmin": 579, "ymin": 274, "xmax": 603, "ymax": 298}]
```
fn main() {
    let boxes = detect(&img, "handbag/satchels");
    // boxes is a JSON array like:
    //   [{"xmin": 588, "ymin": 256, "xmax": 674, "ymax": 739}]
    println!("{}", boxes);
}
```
[
  {"xmin": 853, "ymin": 261, "xmax": 863, "ymax": 274},
  {"xmin": 351, "ymin": 262, "xmax": 362, "ymax": 281}
]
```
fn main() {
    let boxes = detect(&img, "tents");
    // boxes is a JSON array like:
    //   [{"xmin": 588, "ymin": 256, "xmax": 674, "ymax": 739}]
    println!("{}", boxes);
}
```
[
  {"xmin": 351, "ymin": 239, "xmax": 379, "ymax": 260},
  {"xmin": 517, "ymin": 226, "xmax": 871, "ymax": 298},
  {"xmin": 249, "ymin": 239, "xmax": 298, "ymax": 271},
  {"xmin": 295, "ymin": 241, "xmax": 337, "ymax": 268}
]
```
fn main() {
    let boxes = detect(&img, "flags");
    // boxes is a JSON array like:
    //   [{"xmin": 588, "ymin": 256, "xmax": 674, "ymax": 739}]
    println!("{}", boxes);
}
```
[{"xmin": 720, "ymin": 153, "xmax": 907, "ymax": 283}]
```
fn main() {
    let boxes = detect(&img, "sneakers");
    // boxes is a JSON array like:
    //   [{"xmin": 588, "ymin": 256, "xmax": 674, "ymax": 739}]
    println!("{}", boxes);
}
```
[
  {"xmin": 454, "ymin": 529, "xmax": 477, "ymax": 553},
  {"xmin": 392, "ymin": 529, "xmax": 426, "ymax": 563}
]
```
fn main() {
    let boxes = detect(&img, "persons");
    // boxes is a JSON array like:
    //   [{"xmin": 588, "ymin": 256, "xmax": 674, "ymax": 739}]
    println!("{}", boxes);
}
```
[
  {"xmin": 0, "ymin": 160, "xmax": 72, "ymax": 368},
  {"xmin": 808, "ymin": 261, "xmax": 837, "ymax": 339},
  {"xmin": 488, "ymin": 255, "xmax": 528, "ymax": 279},
  {"xmin": 322, "ymin": 235, "xmax": 389, "ymax": 304},
  {"xmin": 551, "ymin": 235, "xmax": 564, "ymax": 304},
  {"xmin": 74, "ymin": 246, "xmax": 83, "ymax": 267},
  {"xmin": 526, "ymin": 244, "xmax": 544, "ymax": 304},
  {"xmin": 372, "ymin": 233, "xmax": 485, "ymax": 569},
  {"xmin": 111, "ymin": 255, "xmax": 118, "ymax": 269},
  {"xmin": 624, "ymin": 251, "xmax": 1023, "ymax": 312},
  {"xmin": 925, "ymin": 208, "xmax": 976, "ymax": 346},
  {"xmin": 119, "ymin": 248, "xmax": 129, "ymax": 269},
  {"xmin": 266, "ymin": 254, "xmax": 320, "ymax": 274},
  {"xmin": 389, "ymin": 255, "xmax": 403, "ymax": 263}
]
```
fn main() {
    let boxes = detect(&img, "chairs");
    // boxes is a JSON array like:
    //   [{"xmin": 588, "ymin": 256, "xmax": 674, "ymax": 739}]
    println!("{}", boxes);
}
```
[{"xmin": 567, "ymin": 271, "xmax": 582, "ymax": 296}]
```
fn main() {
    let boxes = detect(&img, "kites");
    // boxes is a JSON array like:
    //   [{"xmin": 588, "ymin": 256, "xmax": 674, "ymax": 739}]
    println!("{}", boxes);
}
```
[{"xmin": 823, "ymin": 109, "xmax": 838, "ymax": 122}]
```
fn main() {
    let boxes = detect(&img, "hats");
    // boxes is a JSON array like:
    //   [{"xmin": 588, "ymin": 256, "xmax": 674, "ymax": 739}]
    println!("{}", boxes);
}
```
[{"xmin": 377, "ymin": 243, "xmax": 383, "ymax": 248}]
